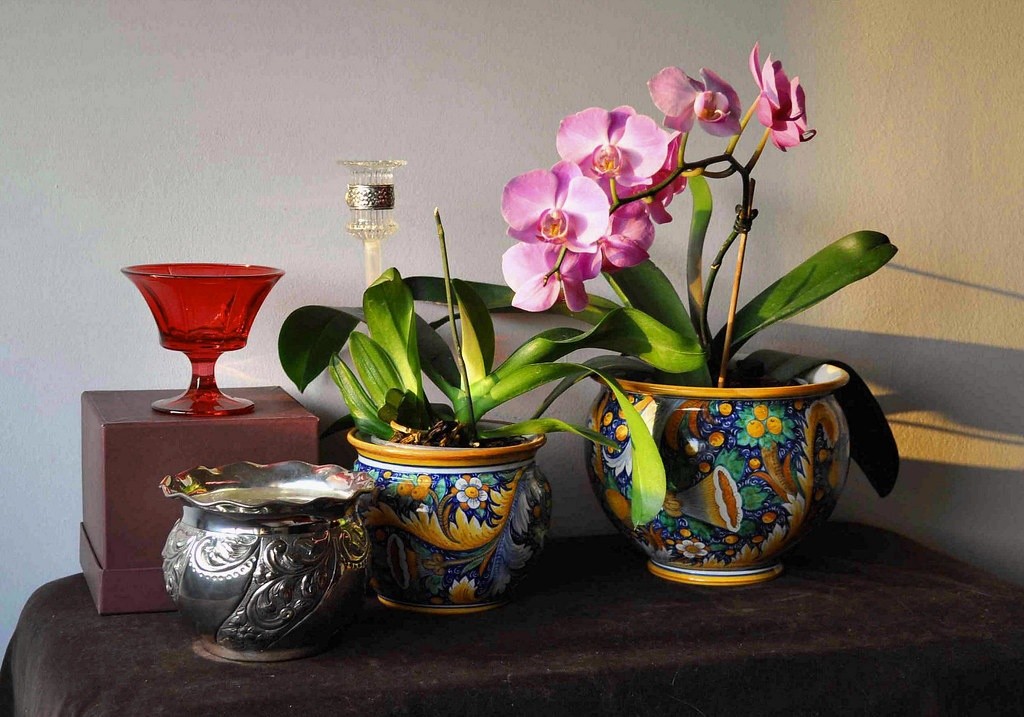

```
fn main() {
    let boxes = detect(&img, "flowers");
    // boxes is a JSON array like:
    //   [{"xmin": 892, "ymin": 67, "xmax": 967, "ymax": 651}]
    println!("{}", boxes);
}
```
[{"xmin": 404, "ymin": 43, "xmax": 903, "ymax": 500}]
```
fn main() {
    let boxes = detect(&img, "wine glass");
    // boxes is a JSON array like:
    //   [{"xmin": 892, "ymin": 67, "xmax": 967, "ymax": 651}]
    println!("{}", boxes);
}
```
[{"xmin": 121, "ymin": 263, "xmax": 285, "ymax": 415}]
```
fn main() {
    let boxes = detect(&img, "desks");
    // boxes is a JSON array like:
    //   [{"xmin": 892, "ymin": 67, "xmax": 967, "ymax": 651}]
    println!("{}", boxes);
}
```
[{"xmin": 0, "ymin": 519, "xmax": 1023, "ymax": 717}]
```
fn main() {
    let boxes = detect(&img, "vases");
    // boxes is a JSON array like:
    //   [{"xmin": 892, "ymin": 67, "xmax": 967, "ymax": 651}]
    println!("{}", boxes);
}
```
[
  {"xmin": 583, "ymin": 351, "xmax": 852, "ymax": 586},
  {"xmin": 120, "ymin": 263, "xmax": 287, "ymax": 416},
  {"xmin": 158, "ymin": 460, "xmax": 377, "ymax": 665}
]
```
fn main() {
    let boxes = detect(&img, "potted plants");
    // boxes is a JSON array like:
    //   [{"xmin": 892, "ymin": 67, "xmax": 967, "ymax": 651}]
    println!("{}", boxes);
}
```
[{"xmin": 278, "ymin": 205, "xmax": 668, "ymax": 616}]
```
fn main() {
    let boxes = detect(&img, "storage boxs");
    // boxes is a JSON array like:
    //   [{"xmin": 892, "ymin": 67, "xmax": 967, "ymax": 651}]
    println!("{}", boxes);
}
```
[{"xmin": 78, "ymin": 386, "xmax": 320, "ymax": 615}]
